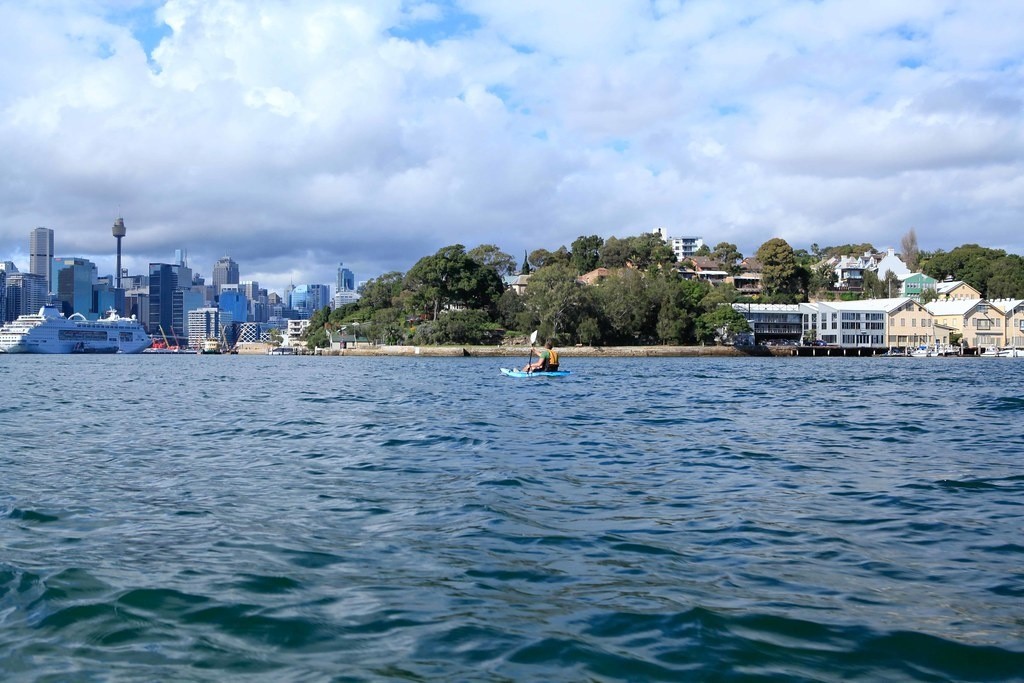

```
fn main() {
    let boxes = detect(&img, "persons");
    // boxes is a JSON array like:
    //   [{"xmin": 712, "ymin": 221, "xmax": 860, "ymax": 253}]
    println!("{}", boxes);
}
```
[{"xmin": 513, "ymin": 340, "xmax": 558, "ymax": 372}]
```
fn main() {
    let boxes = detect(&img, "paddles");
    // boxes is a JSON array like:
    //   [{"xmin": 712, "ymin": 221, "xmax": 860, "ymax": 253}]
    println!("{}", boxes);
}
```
[{"xmin": 527, "ymin": 330, "xmax": 538, "ymax": 373}]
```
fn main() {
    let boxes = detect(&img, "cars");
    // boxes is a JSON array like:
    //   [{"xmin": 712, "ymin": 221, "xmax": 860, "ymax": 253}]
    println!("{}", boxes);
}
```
[
  {"xmin": 815, "ymin": 339, "xmax": 827, "ymax": 346},
  {"xmin": 758, "ymin": 339, "xmax": 801, "ymax": 346}
]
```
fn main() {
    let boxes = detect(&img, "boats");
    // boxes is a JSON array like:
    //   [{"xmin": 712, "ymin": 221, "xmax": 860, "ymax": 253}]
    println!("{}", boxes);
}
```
[
  {"xmin": 910, "ymin": 350, "xmax": 938, "ymax": 357},
  {"xmin": 498, "ymin": 367, "xmax": 577, "ymax": 378},
  {"xmin": 0, "ymin": 303, "xmax": 153, "ymax": 354}
]
